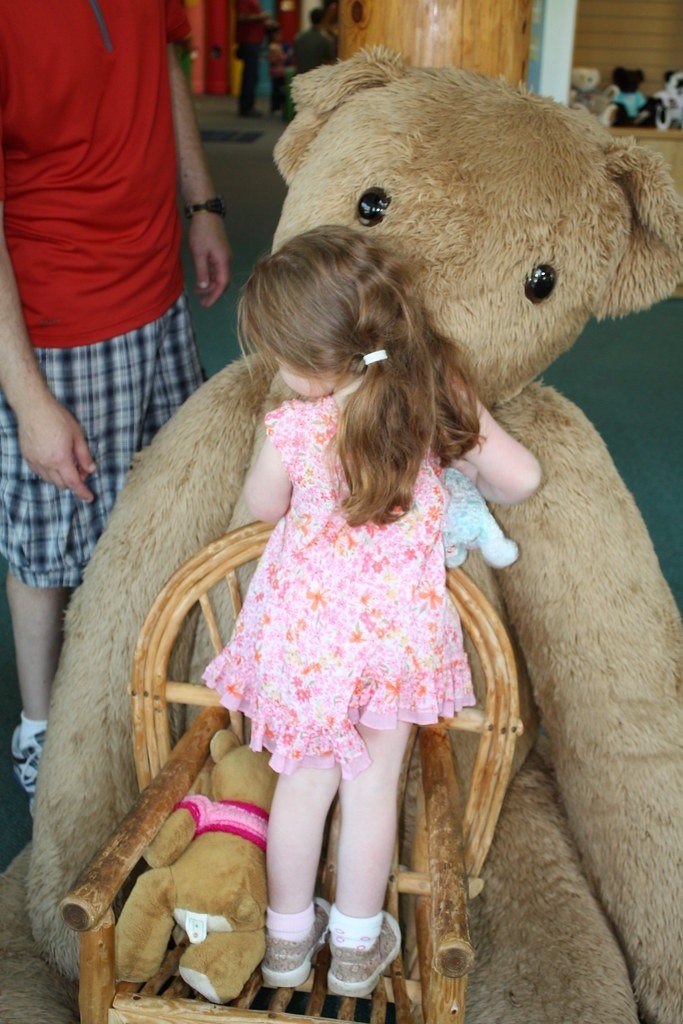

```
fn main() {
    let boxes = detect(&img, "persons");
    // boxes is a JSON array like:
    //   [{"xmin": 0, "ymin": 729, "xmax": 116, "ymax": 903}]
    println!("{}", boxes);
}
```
[
  {"xmin": 231, "ymin": 0, "xmax": 288, "ymax": 119},
  {"xmin": 290, "ymin": 8, "xmax": 336, "ymax": 128},
  {"xmin": 200, "ymin": 222, "xmax": 543, "ymax": 1000},
  {"xmin": 0, "ymin": 1, "xmax": 235, "ymax": 824}
]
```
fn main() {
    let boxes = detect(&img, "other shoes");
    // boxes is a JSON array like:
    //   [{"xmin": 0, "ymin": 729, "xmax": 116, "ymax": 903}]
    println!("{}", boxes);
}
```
[{"xmin": 239, "ymin": 107, "xmax": 262, "ymax": 118}]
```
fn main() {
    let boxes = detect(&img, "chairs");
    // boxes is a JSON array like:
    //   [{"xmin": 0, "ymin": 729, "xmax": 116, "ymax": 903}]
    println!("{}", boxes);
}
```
[{"xmin": 56, "ymin": 513, "xmax": 526, "ymax": 1024}]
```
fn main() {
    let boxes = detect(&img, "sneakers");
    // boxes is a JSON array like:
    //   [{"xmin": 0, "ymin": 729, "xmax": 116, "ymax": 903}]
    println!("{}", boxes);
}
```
[
  {"xmin": 327, "ymin": 909, "xmax": 401, "ymax": 997},
  {"xmin": 11, "ymin": 724, "xmax": 48, "ymax": 819},
  {"xmin": 261, "ymin": 897, "xmax": 331, "ymax": 988}
]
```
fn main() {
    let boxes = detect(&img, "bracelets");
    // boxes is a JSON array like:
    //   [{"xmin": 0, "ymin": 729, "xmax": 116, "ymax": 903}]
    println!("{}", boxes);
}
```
[{"xmin": 185, "ymin": 195, "xmax": 226, "ymax": 218}]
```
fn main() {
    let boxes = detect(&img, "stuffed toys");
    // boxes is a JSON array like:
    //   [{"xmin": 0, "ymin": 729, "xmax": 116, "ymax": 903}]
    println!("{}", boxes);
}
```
[
  {"xmin": 605, "ymin": 66, "xmax": 665, "ymax": 128},
  {"xmin": 655, "ymin": 69, "xmax": 683, "ymax": 130},
  {"xmin": 114, "ymin": 729, "xmax": 288, "ymax": 1005},
  {"xmin": 0, "ymin": 52, "xmax": 683, "ymax": 1024},
  {"xmin": 437, "ymin": 461, "xmax": 520, "ymax": 567},
  {"xmin": 568, "ymin": 67, "xmax": 620, "ymax": 128}
]
